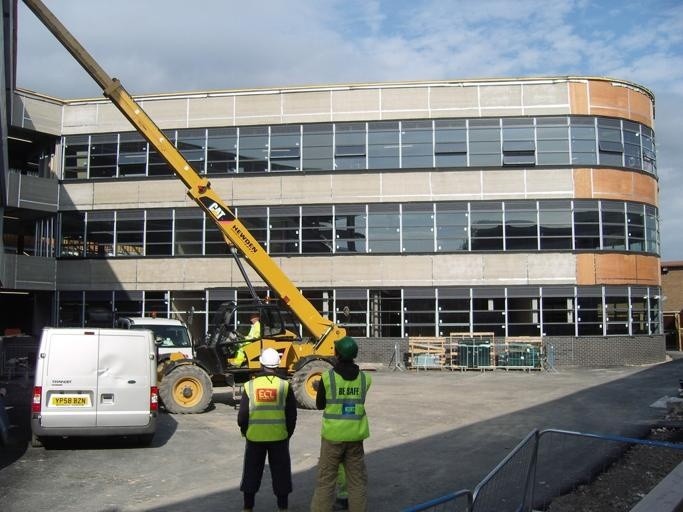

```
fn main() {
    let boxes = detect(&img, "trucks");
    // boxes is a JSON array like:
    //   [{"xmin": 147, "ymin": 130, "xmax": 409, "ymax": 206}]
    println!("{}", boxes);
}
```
[{"xmin": 26, "ymin": 323, "xmax": 161, "ymax": 446}]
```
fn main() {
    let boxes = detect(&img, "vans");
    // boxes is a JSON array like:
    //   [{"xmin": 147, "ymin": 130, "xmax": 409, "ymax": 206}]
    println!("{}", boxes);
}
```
[{"xmin": 117, "ymin": 313, "xmax": 198, "ymax": 368}]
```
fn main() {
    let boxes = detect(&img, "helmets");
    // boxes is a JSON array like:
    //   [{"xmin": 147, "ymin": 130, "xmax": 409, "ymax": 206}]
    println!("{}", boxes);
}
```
[
  {"xmin": 258, "ymin": 346, "xmax": 282, "ymax": 368},
  {"xmin": 331, "ymin": 334, "xmax": 359, "ymax": 358}
]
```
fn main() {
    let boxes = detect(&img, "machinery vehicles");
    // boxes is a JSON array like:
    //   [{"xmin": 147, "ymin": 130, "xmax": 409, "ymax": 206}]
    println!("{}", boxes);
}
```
[{"xmin": 22, "ymin": 0, "xmax": 354, "ymax": 416}]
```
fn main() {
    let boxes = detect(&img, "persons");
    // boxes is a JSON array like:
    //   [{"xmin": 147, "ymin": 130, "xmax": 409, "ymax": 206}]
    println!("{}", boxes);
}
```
[
  {"xmin": 309, "ymin": 335, "xmax": 372, "ymax": 512},
  {"xmin": 237, "ymin": 348, "xmax": 298, "ymax": 512},
  {"xmin": 227, "ymin": 313, "xmax": 261, "ymax": 367}
]
